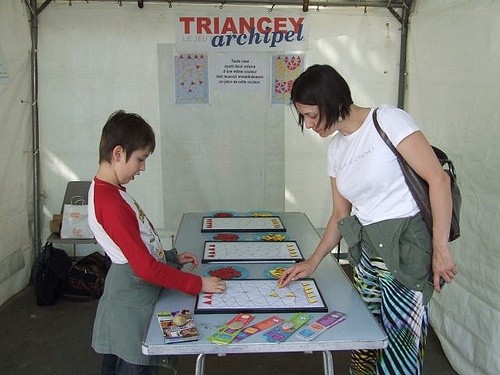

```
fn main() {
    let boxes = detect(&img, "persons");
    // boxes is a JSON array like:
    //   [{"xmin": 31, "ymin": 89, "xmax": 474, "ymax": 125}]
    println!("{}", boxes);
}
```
[
  {"xmin": 279, "ymin": 64, "xmax": 459, "ymax": 375},
  {"xmin": 86, "ymin": 109, "xmax": 225, "ymax": 375}
]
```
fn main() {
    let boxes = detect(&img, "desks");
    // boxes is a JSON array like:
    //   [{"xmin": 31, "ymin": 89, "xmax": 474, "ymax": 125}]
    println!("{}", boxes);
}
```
[{"xmin": 141, "ymin": 206, "xmax": 390, "ymax": 374}]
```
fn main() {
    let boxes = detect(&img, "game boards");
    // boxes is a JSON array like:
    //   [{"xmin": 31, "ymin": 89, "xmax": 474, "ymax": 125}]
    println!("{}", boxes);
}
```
[
  {"xmin": 200, "ymin": 215, "xmax": 287, "ymax": 233},
  {"xmin": 193, "ymin": 278, "xmax": 328, "ymax": 314},
  {"xmin": 202, "ymin": 239, "xmax": 306, "ymax": 265}
]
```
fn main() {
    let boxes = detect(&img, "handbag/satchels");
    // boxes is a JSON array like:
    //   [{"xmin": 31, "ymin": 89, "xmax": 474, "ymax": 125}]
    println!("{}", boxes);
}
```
[
  {"xmin": 59, "ymin": 195, "xmax": 95, "ymax": 240},
  {"xmin": 66, "ymin": 251, "xmax": 112, "ymax": 301},
  {"xmin": 371, "ymin": 107, "xmax": 462, "ymax": 242},
  {"xmin": 31, "ymin": 242, "xmax": 72, "ymax": 303}
]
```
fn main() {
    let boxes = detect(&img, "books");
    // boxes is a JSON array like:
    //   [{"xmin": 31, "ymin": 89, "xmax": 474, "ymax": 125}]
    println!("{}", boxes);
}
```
[{"xmin": 157, "ymin": 309, "xmax": 199, "ymax": 344}]
